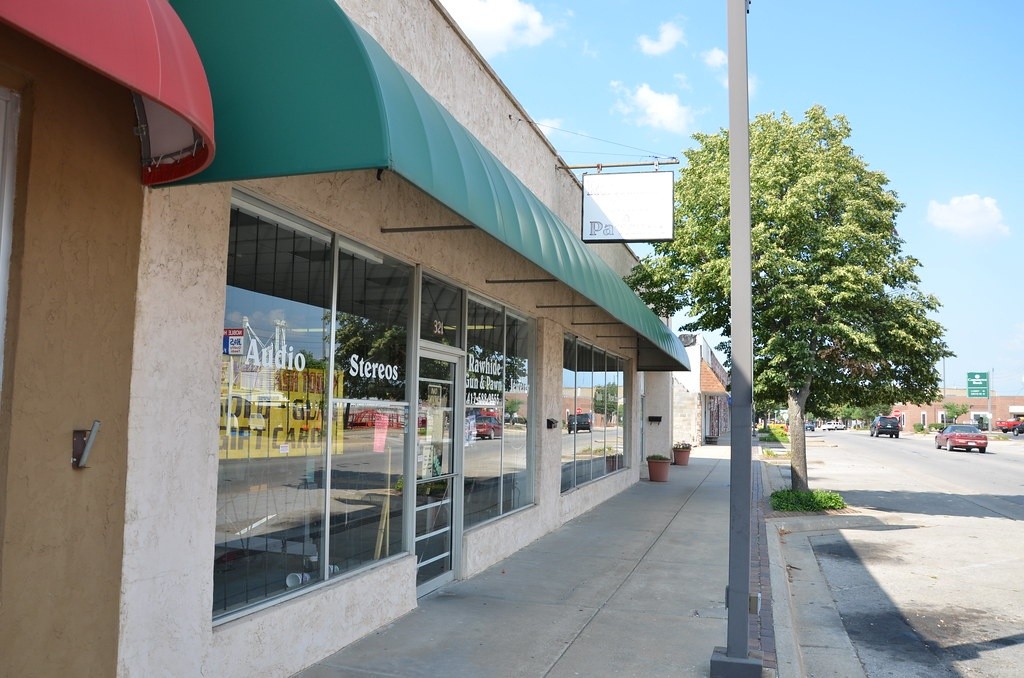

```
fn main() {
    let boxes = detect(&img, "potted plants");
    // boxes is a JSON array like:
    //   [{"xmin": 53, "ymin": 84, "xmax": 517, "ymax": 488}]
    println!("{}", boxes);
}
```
[
  {"xmin": 646, "ymin": 454, "xmax": 671, "ymax": 481},
  {"xmin": 605, "ymin": 453, "xmax": 624, "ymax": 473},
  {"xmin": 706, "ymin": 436, "xmax": 718, "ymax": 445}
]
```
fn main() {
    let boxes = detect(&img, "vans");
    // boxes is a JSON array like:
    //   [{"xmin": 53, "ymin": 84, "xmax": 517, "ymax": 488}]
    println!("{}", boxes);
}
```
[{"xmin": 229, "ymin": 371, "xmax": 283, "ymax": 397}]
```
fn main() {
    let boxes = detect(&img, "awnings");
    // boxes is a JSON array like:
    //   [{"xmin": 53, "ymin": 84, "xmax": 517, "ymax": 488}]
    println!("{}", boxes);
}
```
[
  {"xmin": 155, "ymin": 0, "xmax": 691, "ymax": 372},
  {"xmin": 0, "ymin": 0, "xmax": 215, "ymax": 182}
]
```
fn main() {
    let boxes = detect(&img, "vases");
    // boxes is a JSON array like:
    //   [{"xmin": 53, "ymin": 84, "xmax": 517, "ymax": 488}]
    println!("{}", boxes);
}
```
[{"xmin": 673, "ymin": 448, "xmax": 691, "ymax": 466}]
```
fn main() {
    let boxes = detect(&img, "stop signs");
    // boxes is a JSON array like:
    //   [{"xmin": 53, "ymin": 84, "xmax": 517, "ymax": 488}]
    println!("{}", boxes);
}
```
[{"xmin": 894, "ymin": 410, "xmax": 901, "ymax": 417}]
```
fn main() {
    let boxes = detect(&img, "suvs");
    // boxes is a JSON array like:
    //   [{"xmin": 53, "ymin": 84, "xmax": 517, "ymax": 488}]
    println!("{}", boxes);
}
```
[
  {"xmin": 869, "ymin": 417, "xmax": 899, "ymax": 439},
  {"xmin": 569, "ymin": 413, "xmax": 592, "ymax": 433},
  {"xmin": 826, "ymin": 422, "xmax": 846, "ymax": 431}
]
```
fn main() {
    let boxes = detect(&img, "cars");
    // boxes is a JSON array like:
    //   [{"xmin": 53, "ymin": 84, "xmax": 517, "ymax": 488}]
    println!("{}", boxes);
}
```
[
  {"xmin": 821, "ymin": 424, "xmax": 826, "ymax": 430},
  {"xmin": 804, "ymin": 423, "xmax": 815, "ymax": 431},
  {"xmin": 933, "ymin": 424, "xmax": 988, "ymax": 454},
  {"xmin": 996, "ymin": 419, "xmax": 1024, "ymax": 436},
  {"xmin": 220, "ymin": 393, "xmax": 315, "ymax": 435},
  {"xmin": 469, "ymin": 416, "xmax": 502, "ymax": 440}
]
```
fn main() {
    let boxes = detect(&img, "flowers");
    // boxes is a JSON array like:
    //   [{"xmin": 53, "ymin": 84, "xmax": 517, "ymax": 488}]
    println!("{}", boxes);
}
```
[{"xmin": 673, "ymin": 440, "xmax": 692, "ymax": 449}]
